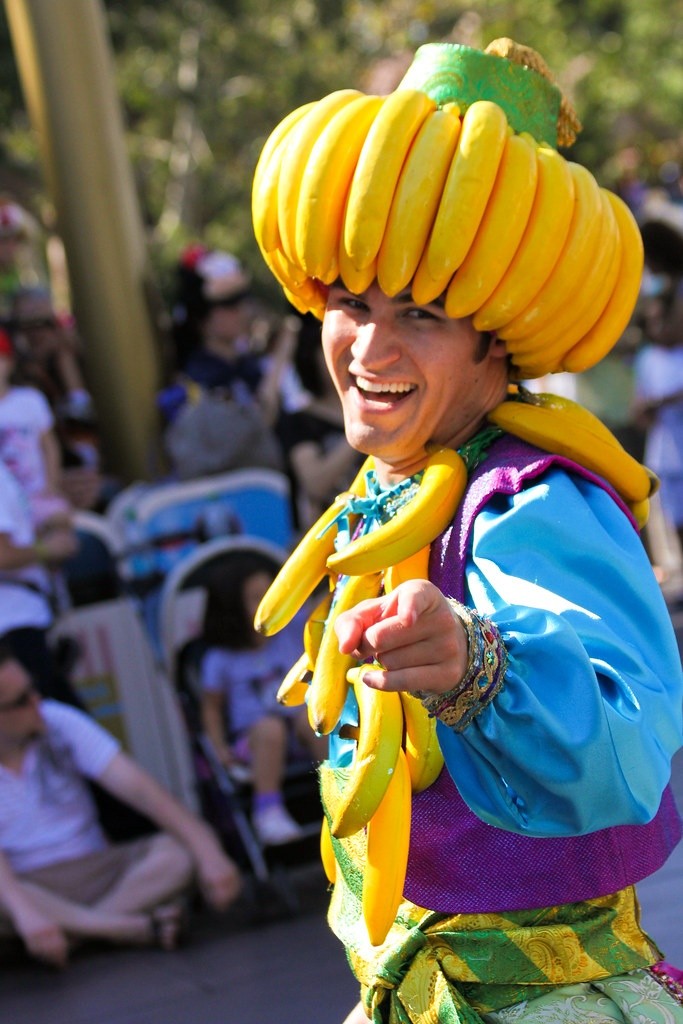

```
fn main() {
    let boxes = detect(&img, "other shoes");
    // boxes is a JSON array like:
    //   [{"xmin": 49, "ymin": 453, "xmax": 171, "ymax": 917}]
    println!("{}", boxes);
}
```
[
  {"xmin": 147, "ymin": 895, "xmax": 189, "ymax": 949},
  {"xmin": 253, "ymin": 803, "xmax": 301, "ymax": 843}
]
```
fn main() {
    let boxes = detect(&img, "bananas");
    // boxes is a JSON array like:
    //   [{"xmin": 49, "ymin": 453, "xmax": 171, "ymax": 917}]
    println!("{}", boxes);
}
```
[
  {"xmin": 485, "ymin": 392, "xmax": 661, "ymax": 530},
  {"xmin": 255, "ymin": 442, "xmax": 468, "ymax": 947}
]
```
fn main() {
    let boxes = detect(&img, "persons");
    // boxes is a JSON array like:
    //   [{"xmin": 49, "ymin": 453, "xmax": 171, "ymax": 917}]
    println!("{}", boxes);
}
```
[
  {"xmin": 255, "ymin": 277, "xmax": 683, "ymax": 1024},
  {"xmin": 0, "ymin": 658, "xmax": 242, "ymax": 959},
  {"xmin": 0, "ymin": 463, "xmax": 160, "ymax": 846},
  {"xmin": 198, "ymin": 557, "xmax": 332, "ymax": 846},
  {"xmin": 0, "ymin": 218, "xmax": 683, "ymax": 607}
]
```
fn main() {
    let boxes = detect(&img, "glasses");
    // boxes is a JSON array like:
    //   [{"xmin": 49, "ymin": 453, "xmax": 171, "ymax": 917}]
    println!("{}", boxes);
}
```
[
  {"xmin": 14, "ymin": 317, "xmax": 58, "ymax": 327},
  {"xmin": 0, "ymin": 684, "xmax": 33, "ymax": 707}
]
